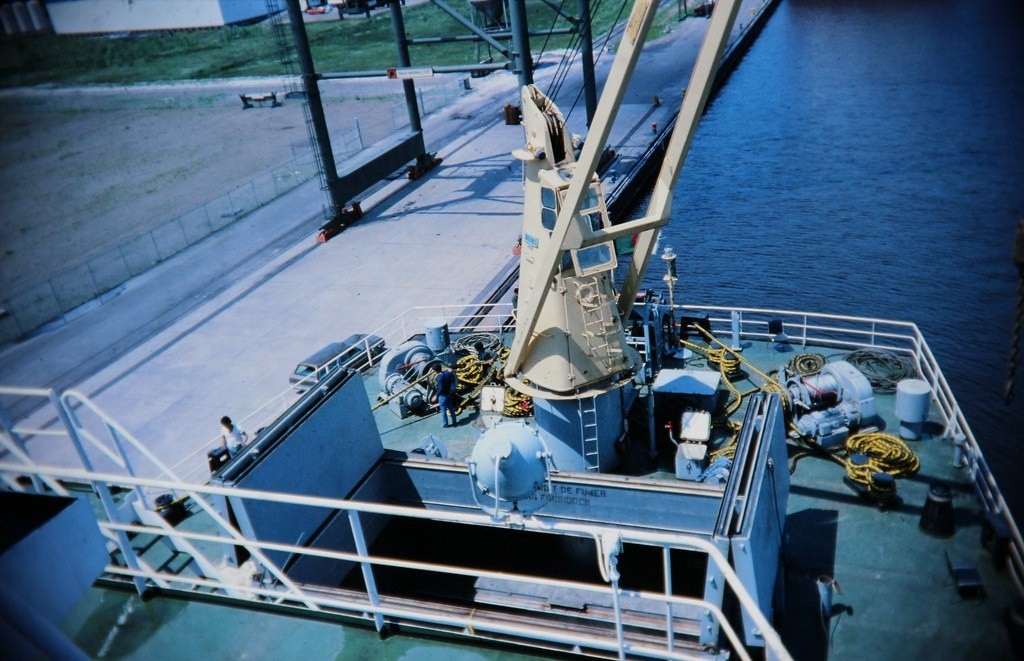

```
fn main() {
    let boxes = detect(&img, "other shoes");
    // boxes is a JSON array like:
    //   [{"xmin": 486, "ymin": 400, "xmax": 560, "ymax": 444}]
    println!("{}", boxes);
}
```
[{"xmin": 441, "ymin": 425, "xmax": 448, "ymax": 428}]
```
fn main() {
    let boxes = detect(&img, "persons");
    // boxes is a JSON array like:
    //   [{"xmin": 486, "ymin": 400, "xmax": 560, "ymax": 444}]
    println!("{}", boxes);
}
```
[
  {"xmin": 474, "ymin": 342, "xmax": 497, "ymax": 386},
  {"xmin": 220, "ymin": 416, "xmax": 248, "ymax": 459},
  {"xmin": 431, "ymin": 364, "xmax": 457, "ymax": 428}
]
